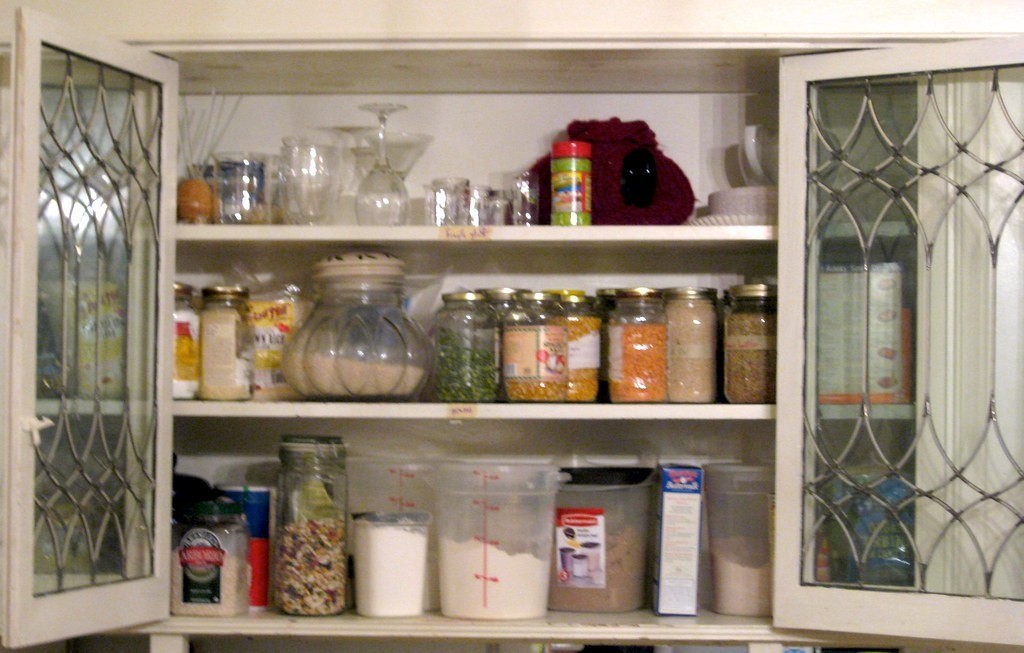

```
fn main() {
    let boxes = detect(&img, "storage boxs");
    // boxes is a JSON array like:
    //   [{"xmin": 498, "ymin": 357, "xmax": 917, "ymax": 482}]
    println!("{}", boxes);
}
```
[{"xmin": 645, "ymin": 462, "xmax": 702, "ymax": 617}]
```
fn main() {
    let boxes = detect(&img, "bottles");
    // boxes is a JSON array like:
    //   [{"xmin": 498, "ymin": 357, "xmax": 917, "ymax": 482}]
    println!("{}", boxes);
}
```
[
  {"xmin": 281, "ymin": 251, "xmax": 433, "ymax": 401},
  {"xmin": 424, "ymin": 171, "xmax": 539, "ymax": 226}
]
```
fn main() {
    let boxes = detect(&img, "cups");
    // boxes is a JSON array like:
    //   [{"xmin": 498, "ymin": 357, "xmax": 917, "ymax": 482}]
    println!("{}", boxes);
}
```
[
  {"xmin": 212, "ymin": 139, "xmax": 339, "ymax": 224},
  {"xmin": 349, "ymin": 511, "xmax": 434, "ymax": 617},
  {"xmin": 702, "ymin": 461, "xmax": 773, "ymax": 614},
  {"xmin": 740, "ymin": 125, "xmax": 778, "ymax": 185}
]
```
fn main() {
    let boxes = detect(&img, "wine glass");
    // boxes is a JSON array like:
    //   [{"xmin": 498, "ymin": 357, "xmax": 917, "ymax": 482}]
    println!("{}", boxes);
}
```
[{"xmin": 351, "ymin": 104, "xmax": 432, "ymax": 226}]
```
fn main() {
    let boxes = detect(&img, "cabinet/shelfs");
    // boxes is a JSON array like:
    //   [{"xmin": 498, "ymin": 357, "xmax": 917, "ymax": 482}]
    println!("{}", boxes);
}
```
[{"xmin": 0, "ymin": 0, "xmax": 1024, "ymax": 653}]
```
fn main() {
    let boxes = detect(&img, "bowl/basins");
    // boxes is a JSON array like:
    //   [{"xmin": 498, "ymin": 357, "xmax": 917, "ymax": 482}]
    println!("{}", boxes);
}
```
[{"xmin": 708, "ymin": 186, "xmax": 777, "ymax": 215}]
